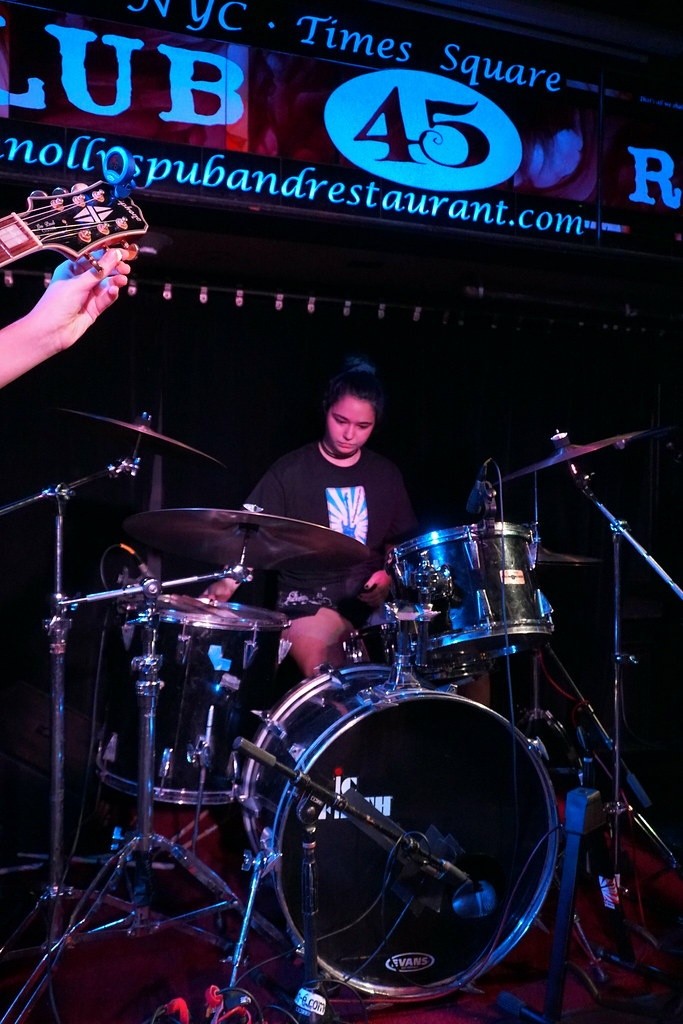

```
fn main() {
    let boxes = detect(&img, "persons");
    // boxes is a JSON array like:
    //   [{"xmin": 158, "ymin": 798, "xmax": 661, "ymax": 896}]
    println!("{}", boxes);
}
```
[
  {"xmin": 198, "ymin": 356, "xmax": 492, "ymax": 717},
  {"xmin": 0, "ymin": 247, "xmax": 130, "ymax": 386}
]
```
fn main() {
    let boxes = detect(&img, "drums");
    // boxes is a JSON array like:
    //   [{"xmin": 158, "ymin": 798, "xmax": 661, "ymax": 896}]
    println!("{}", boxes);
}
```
[
  {"xmin": 384, "ymin": 519, "xmax": 554, "ymax": 683},
  {"xmin": 238, "ymin": 661, "xmax": 563, "ymax": 1004},
  {"xmin": 91, "ymin": 590, "xmax": 288, "ymax": 809}
]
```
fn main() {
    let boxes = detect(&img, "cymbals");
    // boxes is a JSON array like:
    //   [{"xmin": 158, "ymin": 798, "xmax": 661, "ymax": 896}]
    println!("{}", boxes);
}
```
[
  {"xmin": 43, "ymin": 403, "xmax": 232, "ymax": 473},
  {"xmin": 119, "ymin": 501, "xmax": 370, "ymax": 580},
  {"xmin": 492, "ymin": 425, "xmax": 652, "ymax": 487},
  {"xmin": 534, "ymin": 544, "xmax": 607, "ymax": 569}
]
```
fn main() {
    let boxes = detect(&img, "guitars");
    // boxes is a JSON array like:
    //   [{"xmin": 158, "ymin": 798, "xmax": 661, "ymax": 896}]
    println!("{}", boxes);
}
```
[{"xmin": 1, "ymin": 178, "xmax": 150, "ymax": 276}]
